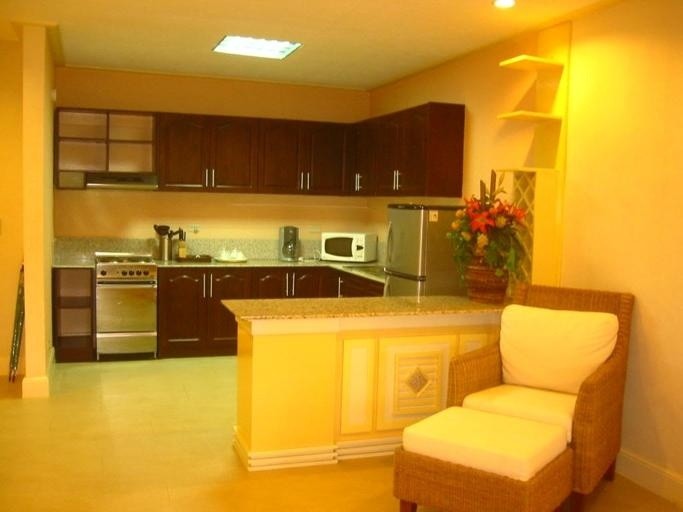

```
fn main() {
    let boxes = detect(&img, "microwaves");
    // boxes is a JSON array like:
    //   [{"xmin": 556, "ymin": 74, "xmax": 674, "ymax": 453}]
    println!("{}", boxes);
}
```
[{"xmin": 319, "ymin": 231, "xmax": 377, "ymax": 263}]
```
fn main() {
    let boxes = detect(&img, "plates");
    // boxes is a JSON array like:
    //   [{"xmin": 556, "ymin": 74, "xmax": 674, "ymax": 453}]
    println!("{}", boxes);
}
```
[{"xmin": 215, "ymin": 257, "xmax": 248, "ymax": 263}]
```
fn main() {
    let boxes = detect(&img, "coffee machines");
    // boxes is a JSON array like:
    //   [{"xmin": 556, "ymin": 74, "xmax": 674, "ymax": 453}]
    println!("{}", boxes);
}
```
[{"xmin": 279, "ymin": 226, "xmax": 299, "ymax": 262}]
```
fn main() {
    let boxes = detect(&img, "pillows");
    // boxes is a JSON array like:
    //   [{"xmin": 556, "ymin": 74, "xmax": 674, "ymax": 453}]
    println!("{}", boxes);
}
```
[{"xmin": 499, "ymin": 303, "xmax": 619, "ymax": 396}]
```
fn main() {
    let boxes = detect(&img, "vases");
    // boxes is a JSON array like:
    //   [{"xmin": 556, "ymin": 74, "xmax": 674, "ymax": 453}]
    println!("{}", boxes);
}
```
[{"xmin": 463, "ymin": 253, "xmax": 508, "ymax": 302}]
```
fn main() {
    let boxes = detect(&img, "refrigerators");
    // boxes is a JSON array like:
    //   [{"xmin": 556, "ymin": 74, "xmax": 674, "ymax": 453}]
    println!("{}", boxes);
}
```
[{"xmin": 384, "ymin": 204, "xmax": 467, "ymax": 297}]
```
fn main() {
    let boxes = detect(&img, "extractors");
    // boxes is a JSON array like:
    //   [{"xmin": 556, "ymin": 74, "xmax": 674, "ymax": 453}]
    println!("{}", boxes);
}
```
[{"xmin": 85, "ymin": 171, "xmax": 158, "ymax": 191}]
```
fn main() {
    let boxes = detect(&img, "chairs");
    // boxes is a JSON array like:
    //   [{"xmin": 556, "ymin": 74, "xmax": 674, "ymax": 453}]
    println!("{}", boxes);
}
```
[{"xmin": 449, "ymin": 285, "xmax": 635, "ymax": 497}]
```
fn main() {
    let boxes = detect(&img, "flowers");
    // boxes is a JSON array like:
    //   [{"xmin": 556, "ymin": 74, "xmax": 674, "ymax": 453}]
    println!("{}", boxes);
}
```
[{"xmin": 446, "ymin": 170, "xmax": 541, "ymax": 282}]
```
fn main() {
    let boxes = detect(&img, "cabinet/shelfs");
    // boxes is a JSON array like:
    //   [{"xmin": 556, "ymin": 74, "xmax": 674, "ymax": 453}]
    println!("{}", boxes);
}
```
[
  {"xmin": 323, "ymin": 267, "xmax": 384, "ymax": 298},
  {"xmin": 52, "ymin": 266, "xmax": 96, "ymax": 364},
  {"xmin": 494, "ymin": 54, "xmax": 565, "ymax": 285},
  {"xmin": 157, "ymin": 266, "xmax": 253, "ymax": 359},
  {"xmin": 254, "ymin": 267, "xmax": 328, "ymax": 300}
]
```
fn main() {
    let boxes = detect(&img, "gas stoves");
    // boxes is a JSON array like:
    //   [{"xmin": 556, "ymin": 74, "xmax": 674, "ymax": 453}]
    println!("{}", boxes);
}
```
[{"xmin": 94, "ymin": 251, "xmax": 153, "ymax": 264}]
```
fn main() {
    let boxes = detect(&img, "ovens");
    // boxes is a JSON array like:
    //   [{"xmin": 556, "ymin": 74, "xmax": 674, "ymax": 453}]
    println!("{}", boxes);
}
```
[{"xmin": 95, "ymin": 265, "xmax": 158, "ymax": 361}]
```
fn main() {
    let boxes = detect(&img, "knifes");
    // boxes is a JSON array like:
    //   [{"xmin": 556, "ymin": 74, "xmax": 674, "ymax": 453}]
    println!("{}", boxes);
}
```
[{"xmin": 178, "ymin": 227, "xmax": 186, "ymax": 242}]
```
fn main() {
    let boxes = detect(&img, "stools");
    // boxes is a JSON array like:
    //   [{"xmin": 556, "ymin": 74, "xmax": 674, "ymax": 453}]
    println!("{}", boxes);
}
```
[{"xmin": 393, "ymin": 407, "xmax": 572, "ymax": 511}]
